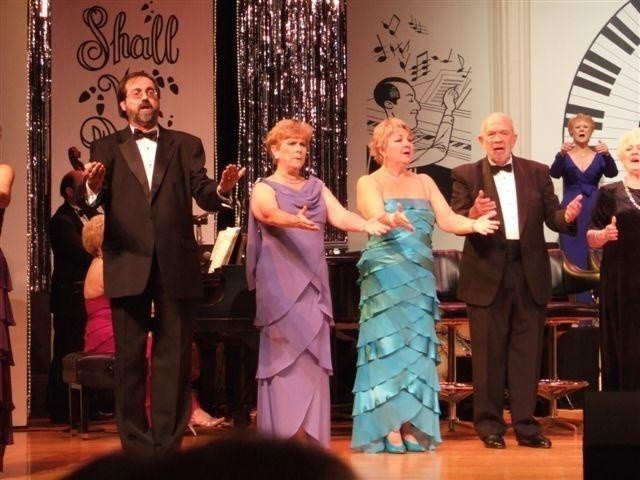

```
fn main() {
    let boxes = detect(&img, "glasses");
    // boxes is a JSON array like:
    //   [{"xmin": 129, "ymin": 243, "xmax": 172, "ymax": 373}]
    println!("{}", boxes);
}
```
[
  {"xmin": 126, "ymin": 89, "xmax": 159, "ymax": 97},
  {"xmin": 485, "ymin": 131, "xmax": 511, "ymax": 139}
]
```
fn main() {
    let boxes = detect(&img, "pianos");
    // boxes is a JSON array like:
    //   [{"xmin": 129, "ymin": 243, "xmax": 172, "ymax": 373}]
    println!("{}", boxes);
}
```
[{"xmin": 193, "ymin": 235, "xmax": 259, "ymax": 418}]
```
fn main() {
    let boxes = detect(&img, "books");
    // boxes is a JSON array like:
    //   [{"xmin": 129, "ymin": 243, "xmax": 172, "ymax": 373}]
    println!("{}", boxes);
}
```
[{"xmin": 208, "ymin": 226, "xmax": 241, "ymax": 274}]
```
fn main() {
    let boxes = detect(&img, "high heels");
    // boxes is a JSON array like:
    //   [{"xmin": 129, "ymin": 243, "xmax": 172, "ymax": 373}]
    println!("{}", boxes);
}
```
[
  {"xmin": 187, "ymin": 413, "xmax": 223, "ymax": 438},
  {"xmin": 407, "ymin": 439, "xmax": 426, "ymax": 452},
  {"xmin": 383, "ymin": 437, "xmax": 405, "ymax": 455}
]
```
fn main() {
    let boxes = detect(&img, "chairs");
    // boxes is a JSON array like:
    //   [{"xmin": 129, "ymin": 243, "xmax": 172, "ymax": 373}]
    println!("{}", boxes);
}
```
[
  {"xmin": 429, "ymin": 248, "xmax": 476, "ymax": 435},
  {"xmin": 537, "ymin": 243, "xmax": 600, "ymax": 435}
]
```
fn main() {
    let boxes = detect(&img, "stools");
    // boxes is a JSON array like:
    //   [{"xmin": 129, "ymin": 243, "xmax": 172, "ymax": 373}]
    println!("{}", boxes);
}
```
[{"xmin": 62, "ymin": 351, "xmax": 116, "ymax": 441}]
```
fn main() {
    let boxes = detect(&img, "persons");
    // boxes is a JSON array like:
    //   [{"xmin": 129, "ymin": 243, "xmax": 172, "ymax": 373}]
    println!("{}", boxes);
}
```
[
  {"xmin": 373, "ymin": 76, "xmax": 459, "ymax": 176},
  {"xmin": 450, "ymin": 112, "xmax": 583, "ymax": 449},
  {"xmin": 81, "ymin": 214, "xmax": 225, "ymax": 437},
  {"xmin": 74, "ymin": 72, "xmax": 247, "ymax": 458},
  {"xmin": 0, "ymin": 124, "xmax": 15, "ymax": 475},
  {"xmin": 245, "ymin": 119, "xmax": 391, "ymax": 451},
  {"xmin": 584, "ymin": 126, "xmax": 639, "ymax": 392},
  {"xmin": 550, "ymin": 113, "xmax": 619, "ymax": 327},
  {"xmin": 45, "ymin": 170, "xmax": 100, "ymax": 425},
  {"xmin": 347, "ymin": 116, "xmax": 501, "ymax": 455}
]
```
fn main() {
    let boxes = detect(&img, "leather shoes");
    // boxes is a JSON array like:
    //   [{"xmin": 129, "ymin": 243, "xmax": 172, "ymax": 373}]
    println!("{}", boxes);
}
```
[
  {"xmin": 485, "ymin": 434, "xmax": 504, "ymax": 448},
  {"xmin": 520, "ymin": 433, "xmax": 551, "ymax": 447}
]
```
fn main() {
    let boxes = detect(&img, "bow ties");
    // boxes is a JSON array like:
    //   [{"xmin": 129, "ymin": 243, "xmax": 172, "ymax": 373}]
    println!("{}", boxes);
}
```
[
  {"xmin": 491, "ymin": 163, "xmax": 513, "ymax": 173},
  {"xmin": 131, "ymin": 129, "xmax": 158, "ymax": 142}
]
